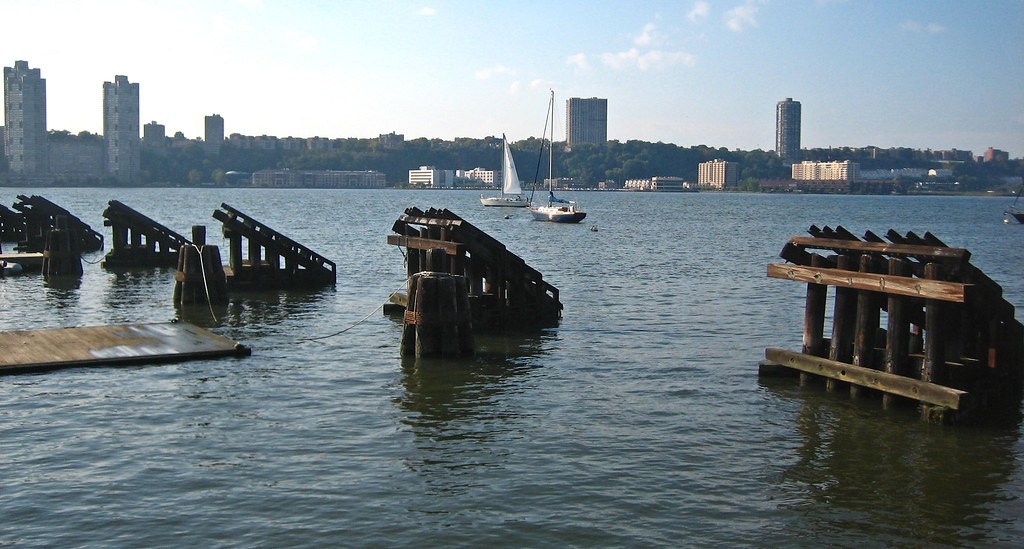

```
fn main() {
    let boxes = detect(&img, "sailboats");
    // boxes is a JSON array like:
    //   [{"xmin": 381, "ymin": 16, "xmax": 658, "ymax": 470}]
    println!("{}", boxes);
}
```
[
  {"xmin": 479, "ymin": 132, "xmax": 531, "ymax": 207},
  {"xmin": 527, "ymin": 89, "xmax": 586, "ymax": 224}
]
